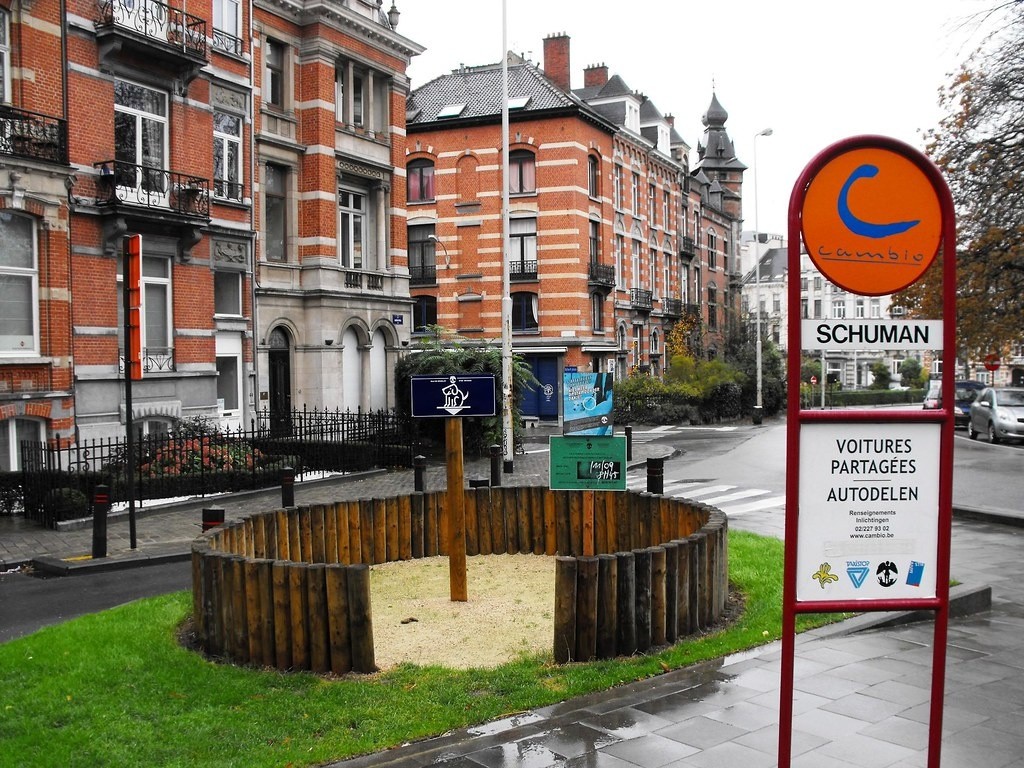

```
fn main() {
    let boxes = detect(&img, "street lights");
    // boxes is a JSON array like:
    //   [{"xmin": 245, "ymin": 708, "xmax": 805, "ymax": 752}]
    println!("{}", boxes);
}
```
[{"xmin": 753, "ymin": 128, "xmax": 774, "ymax": 406}]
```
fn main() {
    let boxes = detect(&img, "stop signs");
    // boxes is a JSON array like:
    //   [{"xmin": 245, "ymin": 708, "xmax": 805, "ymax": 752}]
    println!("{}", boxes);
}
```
[
  {"xmin": 811, "ymin": 377, "xmax": 817, "ymax": 385},
  {"xmin": 984, "ymin": 354, "xmax": 1001, "ymax": 371}
]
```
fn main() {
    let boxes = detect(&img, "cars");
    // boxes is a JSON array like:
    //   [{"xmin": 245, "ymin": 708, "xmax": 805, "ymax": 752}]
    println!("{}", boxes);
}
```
[
  {"xmin": 968, "ymin": 386, "xmax": 1024, "ymax": 444},
  {"xmin": 922, "ymin": 379, "xmax": 987, "ymax": 425}
]
